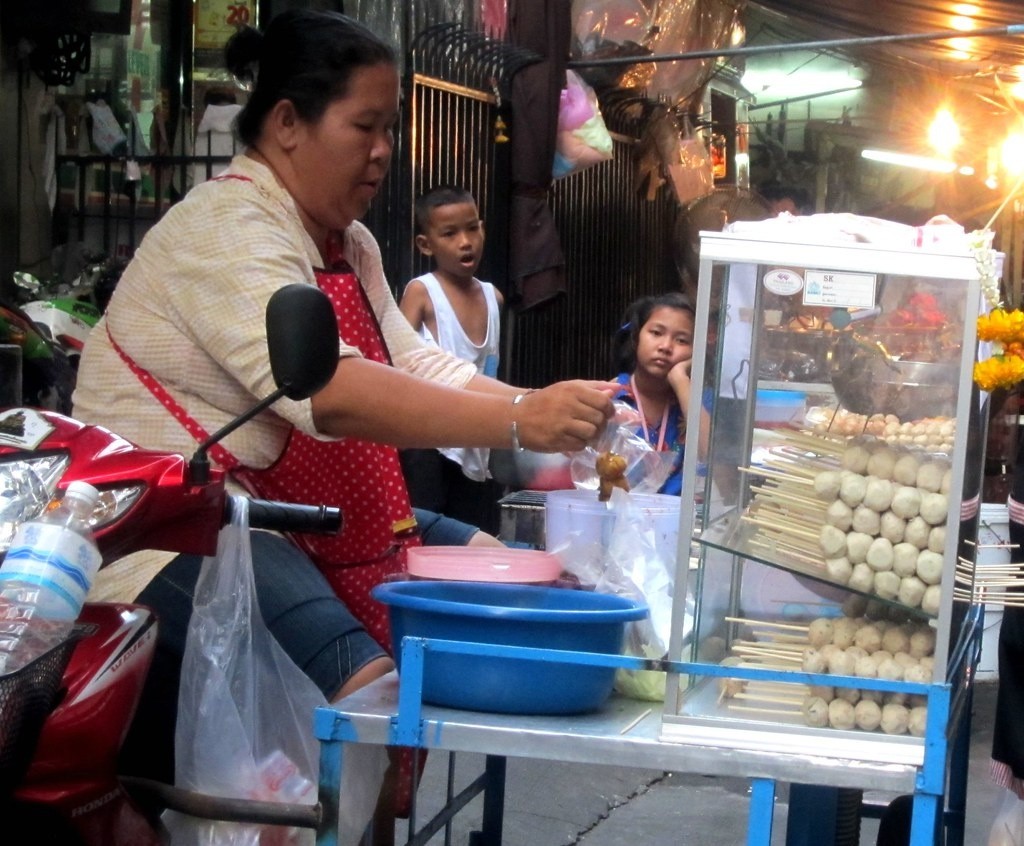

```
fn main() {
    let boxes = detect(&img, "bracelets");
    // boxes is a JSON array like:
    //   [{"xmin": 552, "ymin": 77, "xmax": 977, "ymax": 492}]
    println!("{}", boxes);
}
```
[{"xmin": 510, "ymin": 393, "xmax": 527, "ymax": 452}]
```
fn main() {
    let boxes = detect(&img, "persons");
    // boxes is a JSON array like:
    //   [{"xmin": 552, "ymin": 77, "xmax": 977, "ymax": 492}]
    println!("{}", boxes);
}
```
[
  {"xmin": 590, "ymin": 293, "xmax": 719, "ymax": 502},
  {"xmin": 989, "ymin": 455, "xmax": 1024, "ymax": 799},
  {"xmin": 398, "ymin": 184, "xmax": 506, "ymax": 538},
  {"xmin": 71, "ymin": 8, "xmax": 623, "ymax": 846}
]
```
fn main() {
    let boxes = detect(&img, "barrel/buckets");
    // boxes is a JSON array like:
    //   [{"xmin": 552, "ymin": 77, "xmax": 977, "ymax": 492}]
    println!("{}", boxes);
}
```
[{"xmin": 546, "ymin": 490, "xmax": 697, "ymax": 702}]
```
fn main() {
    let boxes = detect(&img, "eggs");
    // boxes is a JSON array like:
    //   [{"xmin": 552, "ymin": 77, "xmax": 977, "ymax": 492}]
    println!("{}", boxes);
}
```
[{"xmin": 595, "ymin": 453, "xmax": 626, "ymax": 480}]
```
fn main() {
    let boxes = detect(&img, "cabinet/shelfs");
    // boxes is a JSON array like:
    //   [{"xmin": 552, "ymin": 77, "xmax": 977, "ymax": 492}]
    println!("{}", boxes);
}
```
[{"xmin": 660, "ymin": 230, "xmax": 1005, "ymax": 765}]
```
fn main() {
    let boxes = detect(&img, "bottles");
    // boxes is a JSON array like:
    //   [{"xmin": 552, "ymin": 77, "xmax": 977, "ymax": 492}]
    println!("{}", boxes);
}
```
[{"xmin": 0, "ymin": 482, "xmax": 104, "ymax": 675}]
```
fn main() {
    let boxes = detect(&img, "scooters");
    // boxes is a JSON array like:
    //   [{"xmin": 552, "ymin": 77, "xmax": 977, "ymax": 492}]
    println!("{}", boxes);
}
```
[
  {"xmin": 1, "ymin": 282, "xmax": 344, "ymax": 846},
  {"xmin": 0, "ymin": 262, "xmax": 103, "ymax": 419}
]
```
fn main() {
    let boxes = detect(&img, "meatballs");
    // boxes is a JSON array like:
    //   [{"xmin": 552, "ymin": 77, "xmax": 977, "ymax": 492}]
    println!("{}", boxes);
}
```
[{"xmin": 790, "ymin": 406, "xmax": 956, "ymax": 736}]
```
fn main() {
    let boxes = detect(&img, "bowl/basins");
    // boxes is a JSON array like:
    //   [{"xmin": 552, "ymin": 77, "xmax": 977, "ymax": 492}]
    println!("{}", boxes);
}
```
[
  {"xmin": 372, "ymin": 581, "xmax": 646, "ymax": 713},
  {"xmin": 833, "ymin": 361, "xmax": 963, "ymax": 418}
]
